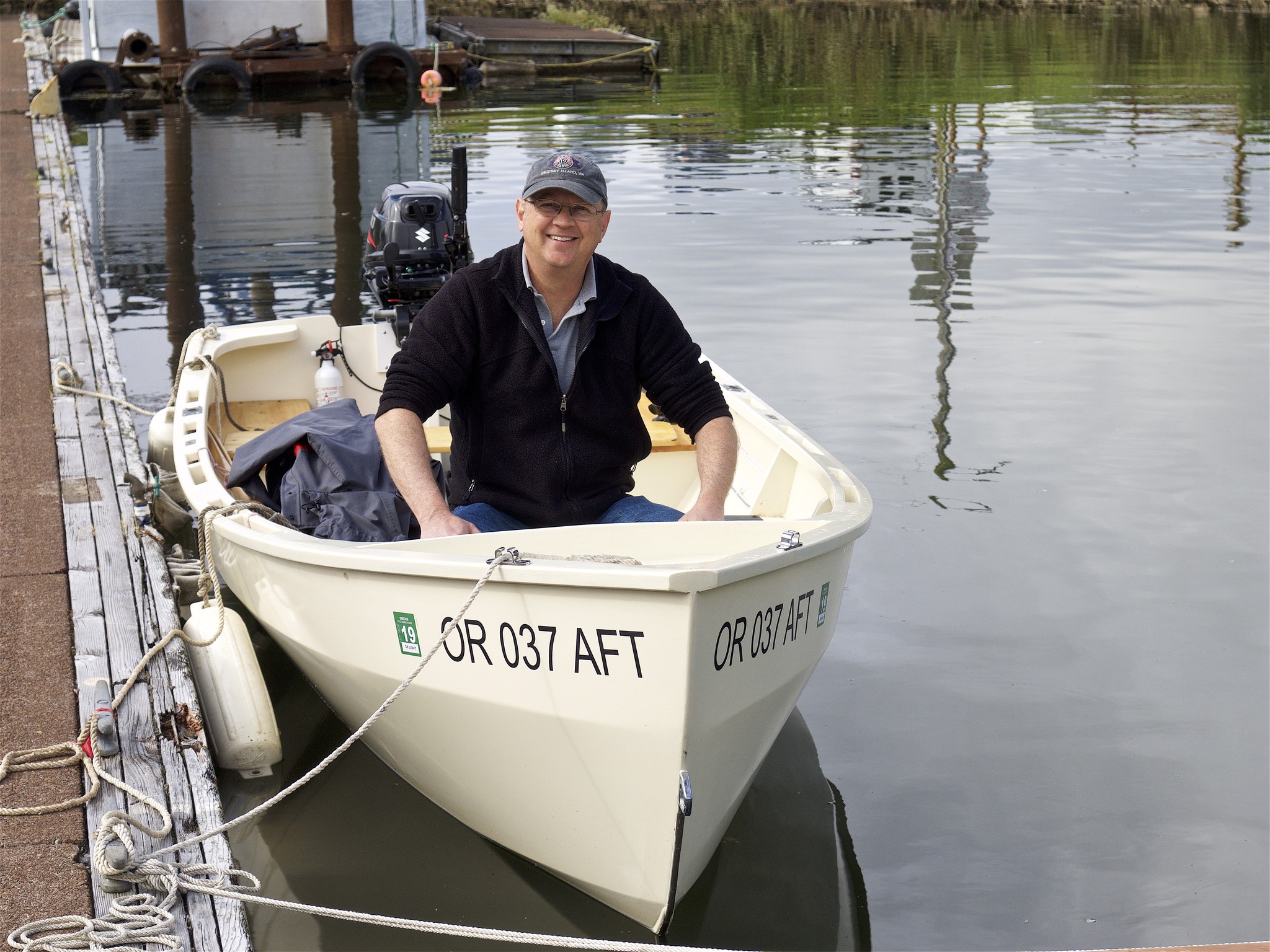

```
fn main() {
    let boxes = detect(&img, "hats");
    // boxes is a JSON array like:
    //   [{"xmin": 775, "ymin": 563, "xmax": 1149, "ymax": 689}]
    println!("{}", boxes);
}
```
[{"xmin": 521, "ymin": 152, "xmax": 608, "ymax": 209}]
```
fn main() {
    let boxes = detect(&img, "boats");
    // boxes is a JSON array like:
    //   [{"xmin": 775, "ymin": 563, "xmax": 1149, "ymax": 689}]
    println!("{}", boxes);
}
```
[{"xmin": 145, "ymin": 181, "xmax": 873, "ymax": 939}]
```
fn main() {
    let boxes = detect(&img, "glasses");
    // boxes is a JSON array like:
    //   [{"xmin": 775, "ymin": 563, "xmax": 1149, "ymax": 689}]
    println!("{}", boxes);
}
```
[{"xmin": 523, "ymin": 198, "xmax": 605, "ymax": 221}]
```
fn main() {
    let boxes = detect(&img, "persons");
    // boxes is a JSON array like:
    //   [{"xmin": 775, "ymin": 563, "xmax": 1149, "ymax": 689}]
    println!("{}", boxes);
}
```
[{"xmin": 372, "ymin": 149, "xmax": 740, "ymax": 541}]
